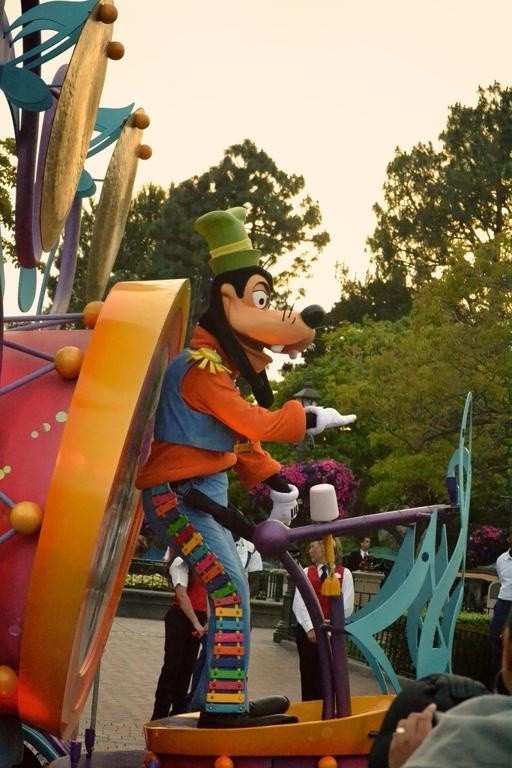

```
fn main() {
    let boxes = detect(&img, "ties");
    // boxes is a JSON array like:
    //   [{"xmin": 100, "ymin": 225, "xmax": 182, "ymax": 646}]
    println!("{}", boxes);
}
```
[{"xmin": 319, "ymin": 564, "xmax": 329, "ymax": 579}]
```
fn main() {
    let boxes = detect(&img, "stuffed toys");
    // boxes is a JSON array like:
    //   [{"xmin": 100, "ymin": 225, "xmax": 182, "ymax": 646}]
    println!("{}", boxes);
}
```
[{"xmin": 133, "ymin": 200, "xmax": 359, "ymax": 733}]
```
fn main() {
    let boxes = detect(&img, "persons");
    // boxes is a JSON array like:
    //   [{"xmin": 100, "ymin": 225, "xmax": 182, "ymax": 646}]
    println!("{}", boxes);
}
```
[
  {"xmin": 149, "ymin": 552, "xmax": 213, "ymax": 721},
  {"xmin": 484, "ymin": 521, "xmax": 512, "ymax": 692},
  {"xmin": 367, "ymin": 603, "xmax": 512, "ymax": 766},
  {"xmin": 291, "ymin": 535, "xmax": 355, "ymax": 700},
  {"xmin": 347, "ymin": 534, "xmax": 376, "ymax": 573},
  {"xmin": 228, "ymin": 506, "xmax": 263, "ymax": 630}
]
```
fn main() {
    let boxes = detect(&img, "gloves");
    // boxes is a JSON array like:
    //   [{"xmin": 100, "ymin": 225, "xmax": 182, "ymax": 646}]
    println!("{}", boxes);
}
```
[{"xmin": 305, "ymin": 402, "xmax": 357, "ymax": 437}]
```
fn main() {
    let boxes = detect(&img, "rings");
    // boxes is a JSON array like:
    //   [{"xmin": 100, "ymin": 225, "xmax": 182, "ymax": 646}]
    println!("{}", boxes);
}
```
[{"xmin": 394, "ymin": 727, "xmax": 405, "ymax": 734}]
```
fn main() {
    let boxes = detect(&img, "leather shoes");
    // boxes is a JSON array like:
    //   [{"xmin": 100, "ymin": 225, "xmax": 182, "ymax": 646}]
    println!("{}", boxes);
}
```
[{"xmin": 196, "ymin": 692, "xmax": 300, "ymax": 730}]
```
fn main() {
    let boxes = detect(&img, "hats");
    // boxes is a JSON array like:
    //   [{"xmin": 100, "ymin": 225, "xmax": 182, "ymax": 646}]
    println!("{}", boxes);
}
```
[{"xmin": 193, "ymin": 205, "xmax": 261, "ymax": 277}]
